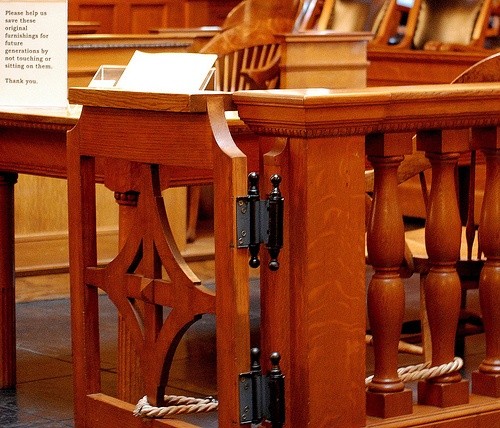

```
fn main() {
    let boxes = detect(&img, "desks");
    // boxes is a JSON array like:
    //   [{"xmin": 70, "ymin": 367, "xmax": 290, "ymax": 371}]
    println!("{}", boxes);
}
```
[{"xmin": 2, "ymin": 102, "xmax": 261, "ymax": 392}]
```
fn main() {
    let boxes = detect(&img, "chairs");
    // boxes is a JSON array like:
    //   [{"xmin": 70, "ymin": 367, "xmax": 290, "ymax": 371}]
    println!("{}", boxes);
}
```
[
  {"xmin": 218, "ymin": 1, "xmax": 499, "ymax": 54},
  {"xmin": 194, "ymin": 17, "xmax": 298, "ymax": 95}
]
